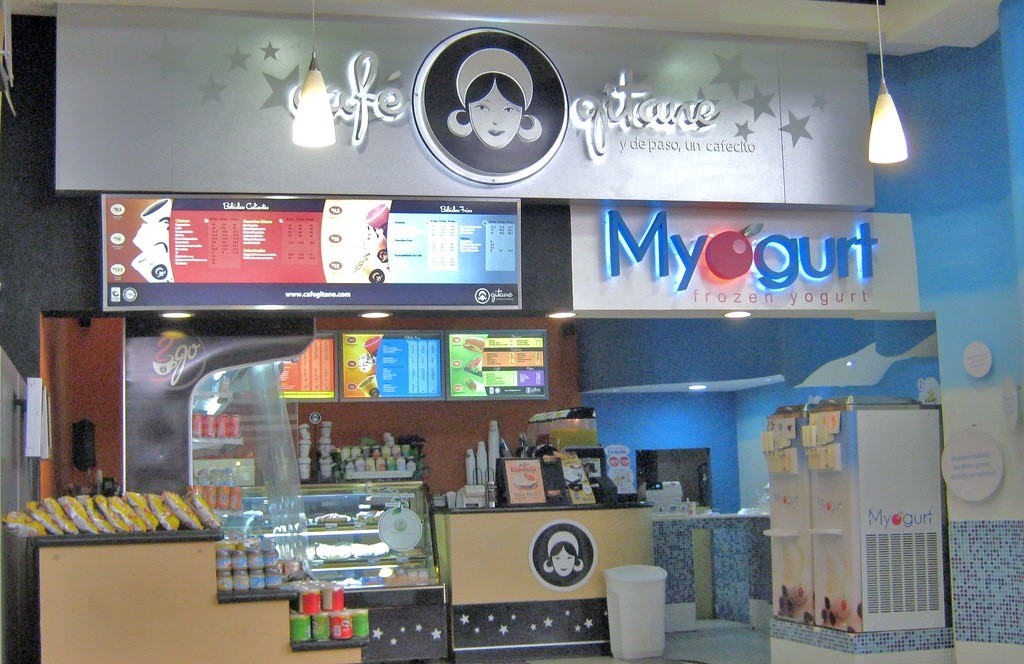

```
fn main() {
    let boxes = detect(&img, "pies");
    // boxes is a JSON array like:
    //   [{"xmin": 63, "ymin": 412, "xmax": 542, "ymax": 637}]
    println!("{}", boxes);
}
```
[{"xmin": 464, "ymin": 378, "xmax": 485, "ymax": 392}]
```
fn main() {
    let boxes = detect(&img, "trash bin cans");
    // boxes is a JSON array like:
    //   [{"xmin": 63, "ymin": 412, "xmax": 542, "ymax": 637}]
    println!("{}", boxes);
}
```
[{"xmin": 603, "ymin": 564, "xmax": 668, "ymax": 660}]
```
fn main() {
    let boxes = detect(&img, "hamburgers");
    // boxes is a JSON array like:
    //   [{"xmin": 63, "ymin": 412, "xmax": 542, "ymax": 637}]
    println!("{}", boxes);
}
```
[{"xmin": 463, "ymin": 338, "xmax": 485, "ymax": 353}]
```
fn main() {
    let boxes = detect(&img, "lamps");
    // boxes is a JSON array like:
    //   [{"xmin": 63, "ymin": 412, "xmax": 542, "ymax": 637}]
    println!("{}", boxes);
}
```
[
  {"xmin": 866, "ymin": 0, "xmax": 909, "ymax": 164},
  {"xmin": 291, "ymin": 1, "xmax": 335, "ymax": 147}
]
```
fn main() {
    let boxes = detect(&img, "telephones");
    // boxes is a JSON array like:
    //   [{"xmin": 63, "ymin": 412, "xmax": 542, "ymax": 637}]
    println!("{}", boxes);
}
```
[{"xmin": 72, "ymin": 418, "xmax": 98, "ymax": 472}]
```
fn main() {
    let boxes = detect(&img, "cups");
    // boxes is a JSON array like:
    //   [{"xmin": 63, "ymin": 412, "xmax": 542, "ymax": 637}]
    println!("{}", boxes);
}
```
[
  {"xmin": 364, "ymin": 336, "xmax": 381, "ymax": 353},
  {"xmin": 129, "ymin": 246, "xmax": 174, "ymax": 284},
  {"xmin": 363, "ymin": 228, "xmax": 389, "ymax": 266},
  {"xmin": 141, "ymin": 198, "xmax": 172, "ymax": 227},
  {"xmin": 355, "ymin": 252, "xmax": 391, "ymax": 284},
  {"xmin": 132, "ymin": 222, "xmax": 170, "ymax": 254},
  {"xmin": 465, "ymin": 420, "xmax": 499, "ymax": 488},
  {"xmin": 358, "ymin": 375, "xmax": 381, "ymax": 399},
  {"xmin": 366, "ymin": 204, "xmax": 390, "ymax": 233}
]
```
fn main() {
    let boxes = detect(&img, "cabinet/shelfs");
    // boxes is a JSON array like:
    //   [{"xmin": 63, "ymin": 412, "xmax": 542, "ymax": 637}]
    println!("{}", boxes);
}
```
[{"xmin": 216, "ymin": 480, "xmax": 440, "ymax": 591}]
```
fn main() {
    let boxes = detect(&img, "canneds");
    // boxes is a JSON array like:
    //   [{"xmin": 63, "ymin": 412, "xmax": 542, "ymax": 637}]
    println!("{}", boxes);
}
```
[
  {"xmin": 288, "ymin": 584, "xmax": 370, "ymax": 641},
  {"xmin": 340, "ymin": 444, "xmax": 416, "ymax": 473},
  {"xmin": 193, "ymin": 484, "xmax": 242, "ymax": 511},
  {"xmin": 216, "ymin": 549, "xmax": 281, "ymax": 592},
  {"xmin": 192, "ymin": 413, "xmax": 240, "ymax": 438}
]
